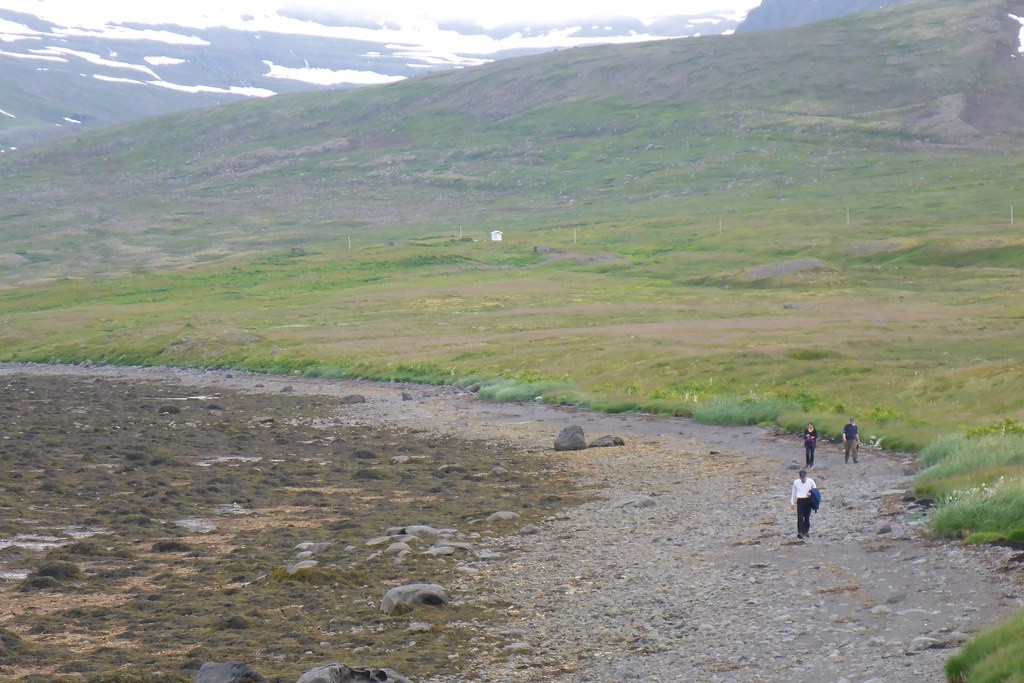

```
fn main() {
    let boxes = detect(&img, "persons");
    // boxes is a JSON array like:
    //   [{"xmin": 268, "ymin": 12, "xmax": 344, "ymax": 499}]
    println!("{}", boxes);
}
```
[
  {"xmin": 804, "ymin": 423, "xmax": 817, "ymax": 468},
  {"xmin": 791, "ymin": 470, "xmax": 816, "ymax": 538},
  {"xmin": 843, "ymin": 417, "xmax": 859, "ymax": 463}
]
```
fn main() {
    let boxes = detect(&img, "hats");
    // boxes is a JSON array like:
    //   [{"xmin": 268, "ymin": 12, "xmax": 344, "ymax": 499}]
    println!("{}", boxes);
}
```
[{"xmin": 800, "ymin": 470, "xmax": 807, "ymax": 476}]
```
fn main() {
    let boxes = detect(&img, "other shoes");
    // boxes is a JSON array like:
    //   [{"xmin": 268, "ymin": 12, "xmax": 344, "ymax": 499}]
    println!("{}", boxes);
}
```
[
  {"xmin": 804, "ymin": 532, "xmax": 810, "ymax": 538},
  {"xmin": 797, "ymin": 533, "xmax": 806, "ymax": 540},
  {"xmin": 854, "ymin": 460, "xmax": 858, "ymax": 463}
]
